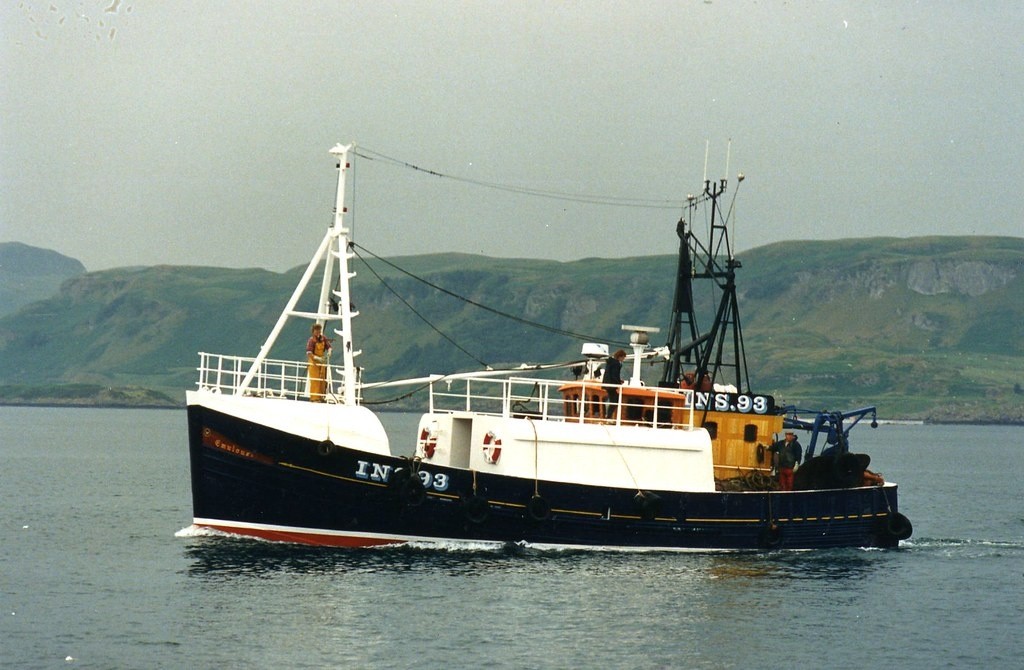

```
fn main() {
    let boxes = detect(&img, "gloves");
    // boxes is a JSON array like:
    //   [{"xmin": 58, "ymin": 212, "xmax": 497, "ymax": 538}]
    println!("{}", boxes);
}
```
[
  {"xmin": 326, "ymin": 347, "xmax": 332, "ymax": 360},
  {"xmin": 762, "ymin": 444, "xmax": 769, "ymax": 450},
  {"xmin": 793, "ymin": 461, "xmax": 799, "ymax": 473},
  {"xmin": 313, "ymin": 355, "xmax": 325, "ymax": 364}
]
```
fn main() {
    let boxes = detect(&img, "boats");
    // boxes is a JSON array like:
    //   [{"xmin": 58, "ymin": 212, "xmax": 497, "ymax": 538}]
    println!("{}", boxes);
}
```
[{"xmin": 173, "ymin": 137, "xmax": 915, "ymax": 558}]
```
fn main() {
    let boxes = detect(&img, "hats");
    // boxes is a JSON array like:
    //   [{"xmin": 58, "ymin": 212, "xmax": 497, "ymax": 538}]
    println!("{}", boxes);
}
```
[{"xmin": 785, "ymin": 429, "xmax": 794, "ymax": 434}]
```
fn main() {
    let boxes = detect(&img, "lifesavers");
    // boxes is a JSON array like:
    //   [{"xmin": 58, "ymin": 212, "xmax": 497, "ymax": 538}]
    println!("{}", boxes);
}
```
[
  {"xmin": 317, "ymin": 440, "xmax": 335, "ymax": 459},
  {"xmin": 529, "ymin": 495, "xmax": 554, "ymax": 522},
  {"xmin": 481, "ymin": 431, "xmax": 503, "ymax": 465},
  {"xmin": 760, "ymin": 524, "xmax": 783, "ymax": 547},
  {"xmin": 638, "ymin": 492, "xmax": 662, "ymax": 521},
  {"xmin": 419, "ymin": 426, "xmax": 438, "ymax": 458},
  {"xmin": 885, "ymin": 511, "xmax": 913, "ymax": 540},
  {"xmin": 756, "ymin": 443, "xmax": 766, "ymax": 463},
  {"xmin": 459, "ymin": 489, "xmax": 492, "ymax": 524},
  {"xmin": 396, "ymin": 475, "xmax": 429, "ymax": 510}
]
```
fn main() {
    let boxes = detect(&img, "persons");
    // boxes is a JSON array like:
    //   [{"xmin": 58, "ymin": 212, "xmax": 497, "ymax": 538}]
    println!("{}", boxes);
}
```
[
  {"xmin": 763, "ymin": 432, "xmax": 802, "ymax": 490},
  {"xmin": 601, "ymin": 349, "xmax": 626, "ymax": 418},
  {"xmin": 305, "ymin": 324, "xmax": 331, "ymax": 402}
]
